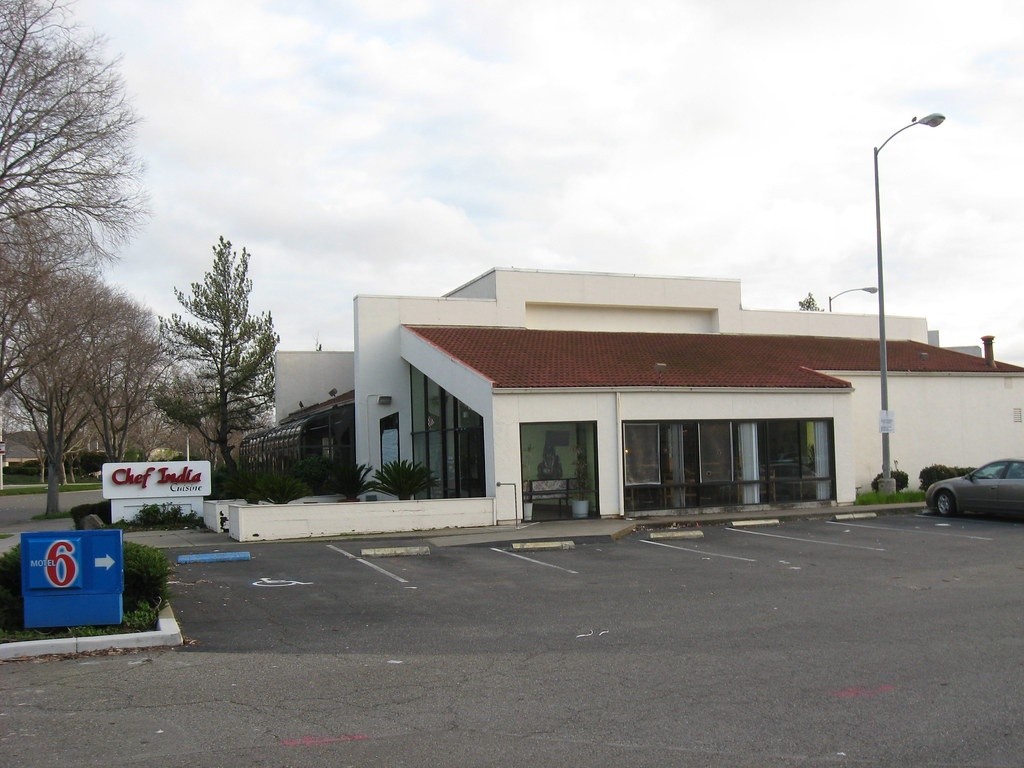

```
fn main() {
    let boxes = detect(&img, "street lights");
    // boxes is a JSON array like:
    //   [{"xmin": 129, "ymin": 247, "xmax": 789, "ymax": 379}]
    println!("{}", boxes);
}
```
[
  {"xmin": 877, "ymin": 112, "xmax": 946, "ymax": 496},
  {"xmin": 829, "ymin": 287, "xmax": 879, "ymax": 313}
]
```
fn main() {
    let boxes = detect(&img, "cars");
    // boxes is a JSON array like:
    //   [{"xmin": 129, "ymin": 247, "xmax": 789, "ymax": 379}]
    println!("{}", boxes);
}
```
[{"xmin": 925, "ymin": 457, "xmax": 1024, "ymax": 521}]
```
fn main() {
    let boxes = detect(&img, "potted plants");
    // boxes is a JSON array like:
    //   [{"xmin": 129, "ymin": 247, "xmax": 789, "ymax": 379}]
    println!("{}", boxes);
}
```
[
  {"xmin": 523, "ymin": 443, "xmax": 533, "ymax": 520},
  {"xmin": 569, "ymin": 446, "xmax": 589, "ymax": 519}
]
